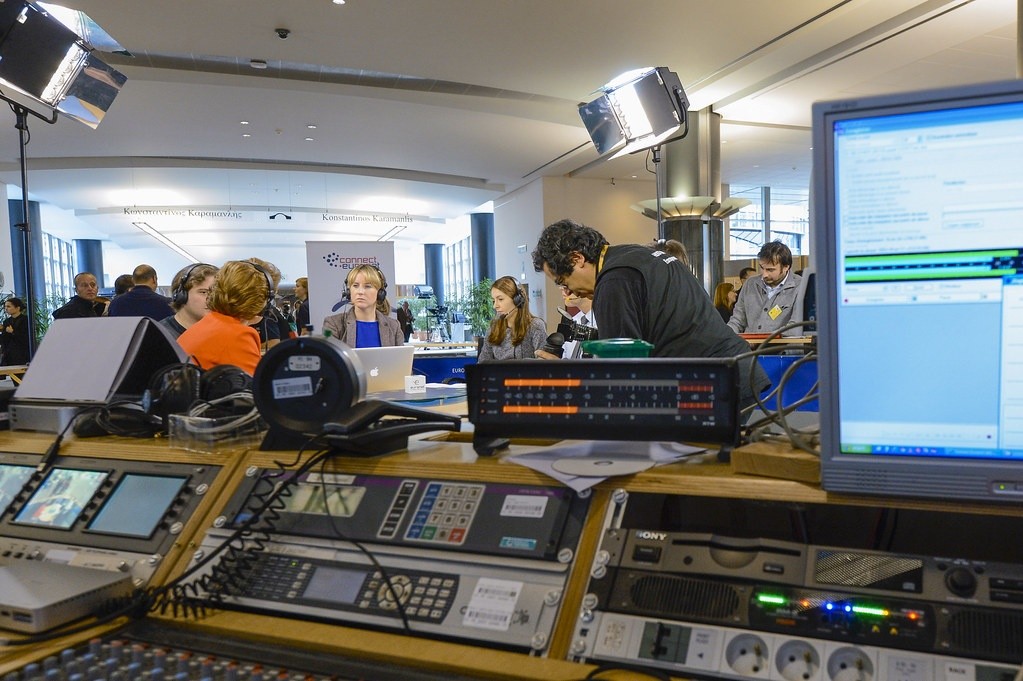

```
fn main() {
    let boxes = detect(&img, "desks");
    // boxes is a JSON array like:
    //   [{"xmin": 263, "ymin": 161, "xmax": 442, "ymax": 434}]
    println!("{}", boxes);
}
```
[{"xmin": 412, "ymin": 343, "xmax": 479, "ymax": 350}]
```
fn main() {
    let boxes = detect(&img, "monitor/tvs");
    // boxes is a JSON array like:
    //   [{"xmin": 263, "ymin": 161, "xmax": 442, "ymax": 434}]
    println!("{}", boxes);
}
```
[
  {"xmin": 812, "ymin": 77, "xmax": 1023, "ymax": 507},
  {"xmin": 0, "ymin": 463, "xmax": 193, "ymax": 540}
]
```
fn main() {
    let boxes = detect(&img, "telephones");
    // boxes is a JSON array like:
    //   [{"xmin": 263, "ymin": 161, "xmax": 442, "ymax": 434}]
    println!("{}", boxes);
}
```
[{"xmin": 321, "ymin": 399, "xmax": 462, "ymax": 458}]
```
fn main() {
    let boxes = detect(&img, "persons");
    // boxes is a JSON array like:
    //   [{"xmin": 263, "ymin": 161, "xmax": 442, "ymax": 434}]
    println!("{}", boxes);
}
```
[
  {"xmin": 108, "ymin": 274, "xmax": 134, "ymax": 312},
  {"xmin": 0, "ymin": 298, "xmax": 30, "ymax": 387},
  {"xmin": 95, "ymin": 297, "xmax": 111, "ymax": 317},
  {"xmin": 397, "ymin": 302, "xmax": 415, "ymax": 342},
  {"xmin": 158, "ymin": 263, "xmax": 220, "ymax": 340},
  {"xmin": 322, "ymin": 263, "xmax": 405, "ymax": 348},
  {"xmin": 108, "ymin": 264, "xmax": 176, "ymax": 322},
  {"xmin": 558, "ymin": 284, "xmax": 598, "ymax": 340},
  {"xmin": 726, "ymin": 240, "xmax": 805, "ymax": 355},
  {"xmin": 714, "ymin": 282, "xmax": 737, "ymax": 324},
  {"xmin": 531, "ymin": 218, "xmax": 772, "ymax": 425},
  {"xmin": 51, "ymin": 273, "xmax": 106, "ymax": 319},
  {"xmin": 732, "ymin": 267, "xmax": 756, "ymax": 306},
  {"xmin": 477, "ymin": 276, "xmax": 547, "ymax": 364},
  {"xmin": 177, "ymin": 257, "xmax": 310, "ymax": 379}
]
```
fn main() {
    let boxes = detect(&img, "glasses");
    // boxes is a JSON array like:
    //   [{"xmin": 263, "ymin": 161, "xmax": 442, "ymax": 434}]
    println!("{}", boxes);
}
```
[{"xmin": 555, "ymin": 275, "xmax": 568, "ymax": 289}]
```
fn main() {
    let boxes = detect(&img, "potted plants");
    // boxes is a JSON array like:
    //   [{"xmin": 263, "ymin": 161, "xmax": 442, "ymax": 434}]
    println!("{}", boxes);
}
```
[
  {"xmin": 400, "ymin": 298, "xmax": 435, "ymax": 340},
  {"xmin": 462, "ymin": 278, "xmax": 496, "ymax": 345}
]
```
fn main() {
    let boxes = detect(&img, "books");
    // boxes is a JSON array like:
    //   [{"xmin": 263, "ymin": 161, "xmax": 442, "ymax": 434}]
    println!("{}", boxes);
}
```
[{"xmin": 736, "ymin": 333, "xmax": 784, "ymax": 338}]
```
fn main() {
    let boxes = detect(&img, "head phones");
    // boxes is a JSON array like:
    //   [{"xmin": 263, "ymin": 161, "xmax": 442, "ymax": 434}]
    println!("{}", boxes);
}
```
[
  {"xmin": 241, "ymin": 260, "xmax": 277, "ymax": 318},
  {"xmin": 503, "ymin": 276, "xmax": 526, "ymax": 309},
  {"xmin": 173, "ymin": 263, "xmax": 211, "ymax": 305},
  {"xmin": 146, "ymin": 363, "xmax": 256, "ymax": 419},
  {"xmin": 342, "ymin": 266, "xmax": 387, "ymax": 304}
]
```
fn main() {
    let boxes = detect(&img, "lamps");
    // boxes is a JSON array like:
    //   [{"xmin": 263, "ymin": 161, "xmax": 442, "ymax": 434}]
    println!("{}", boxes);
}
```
[
  {"xmin": 1, "ymin": 0, "xmax": 136, "ymax": 365},
  {"xmin": 579, "ymin": 64, "xmax": 690, "ymax": 239}
]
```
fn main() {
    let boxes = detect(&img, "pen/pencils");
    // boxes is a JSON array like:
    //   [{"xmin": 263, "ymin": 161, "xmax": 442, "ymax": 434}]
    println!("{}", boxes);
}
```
[{"xmin": 782, "ymin": 306, "xmax": 788, "ymax": 309}]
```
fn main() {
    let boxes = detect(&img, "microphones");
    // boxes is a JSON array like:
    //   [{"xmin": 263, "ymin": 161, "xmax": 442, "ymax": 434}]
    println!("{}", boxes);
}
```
[{"xmin": 500, "ymin": 302, "xmax": 520, "ymax": 320}]
